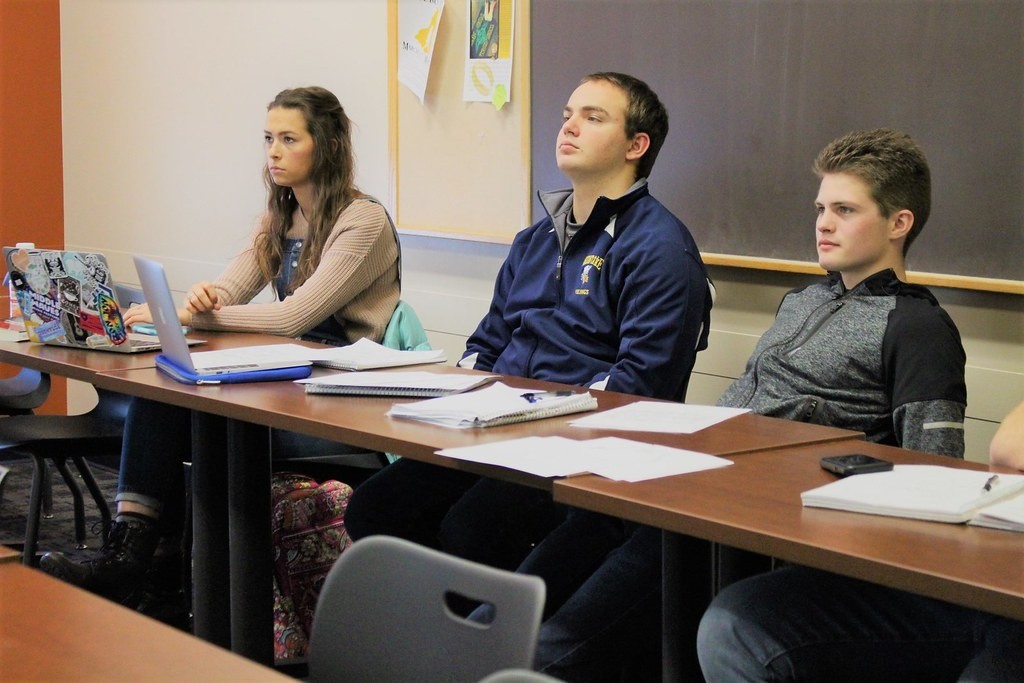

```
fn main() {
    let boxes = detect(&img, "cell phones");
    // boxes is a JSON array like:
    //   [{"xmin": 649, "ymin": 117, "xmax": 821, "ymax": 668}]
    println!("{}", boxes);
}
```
[
  {"xmin": 820, "ymin": 453, "xmax": 893, "ymax": 477},
  {"xmin": 134, "ymin": 323, "xmax": 191, "ymax": 335}
]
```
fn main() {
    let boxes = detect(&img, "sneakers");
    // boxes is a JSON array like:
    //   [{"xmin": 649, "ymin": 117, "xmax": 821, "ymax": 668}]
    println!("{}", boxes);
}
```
[{"xmin": 39, "ymin": 517, "xmax": 160, "ymax": 606}]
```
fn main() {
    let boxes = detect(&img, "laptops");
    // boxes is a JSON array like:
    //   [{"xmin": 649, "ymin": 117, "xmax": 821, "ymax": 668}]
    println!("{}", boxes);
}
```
[
  {"xmin": 3, "ymin": 246, "xmax": 208, "ymax": 353},
  {"xmin": 132, "ymin": 256, "xmax": 313, "ymax": 375}
]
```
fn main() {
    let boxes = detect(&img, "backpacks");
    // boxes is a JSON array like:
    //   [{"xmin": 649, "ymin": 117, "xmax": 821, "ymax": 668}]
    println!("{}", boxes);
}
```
[{"xmin": 267, "ymin": 471, "xmax": 357, "ymax": 666}]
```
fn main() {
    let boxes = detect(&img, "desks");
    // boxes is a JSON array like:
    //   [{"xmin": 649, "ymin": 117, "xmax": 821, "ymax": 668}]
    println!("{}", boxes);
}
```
[
  {"xmin": 0, "ymin": 330, "xmax": 1024, "ymax": 683},
  {"xmin": 0, "ymin": 563, "xmax": 307, "ymax": 683}
]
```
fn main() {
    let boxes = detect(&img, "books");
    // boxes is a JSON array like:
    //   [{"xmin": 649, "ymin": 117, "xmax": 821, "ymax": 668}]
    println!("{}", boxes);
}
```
[{"xmin": 293, "ymin": 338, "xmax": 1023, "ymax": 533}]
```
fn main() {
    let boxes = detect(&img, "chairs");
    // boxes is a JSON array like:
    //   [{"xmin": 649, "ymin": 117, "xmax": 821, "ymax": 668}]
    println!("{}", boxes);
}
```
[
  {"xmin": 0, "ymin": 384, "xmax": 133, "ymax": 568},
  {"xmin": 307, "ymin": 535, "xmax": 546, "ymax": 683},
  {"xmin": 0, "ymin": 367, "xmax": 54, "ymax": 517}
]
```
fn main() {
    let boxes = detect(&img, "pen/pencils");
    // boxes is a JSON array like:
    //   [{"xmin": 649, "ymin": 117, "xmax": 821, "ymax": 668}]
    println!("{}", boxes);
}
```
[
  {"xmin": 522, "ymin": 390, "xmax": 576, "ymax": 400},
  {"xmin": 980, "ymin": 475, "xmax": 999, "ymax": 492}
]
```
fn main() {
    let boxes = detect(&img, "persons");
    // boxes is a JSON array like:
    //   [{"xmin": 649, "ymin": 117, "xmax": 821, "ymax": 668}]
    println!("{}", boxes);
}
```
[
  {"xmin": 695, "ymin": 398, "xmax": 1023, "ymax": 683},
  {"xmin": 345, "ymin": 74, "xmax": 712, "ymax": 618},
  {"xmin": 39, "ymin": 86, "xmax": 401, "ymax": 605},
  {"xmin": 463, "ymin": 130, "xmax": 968, "ymax": 683}
]
